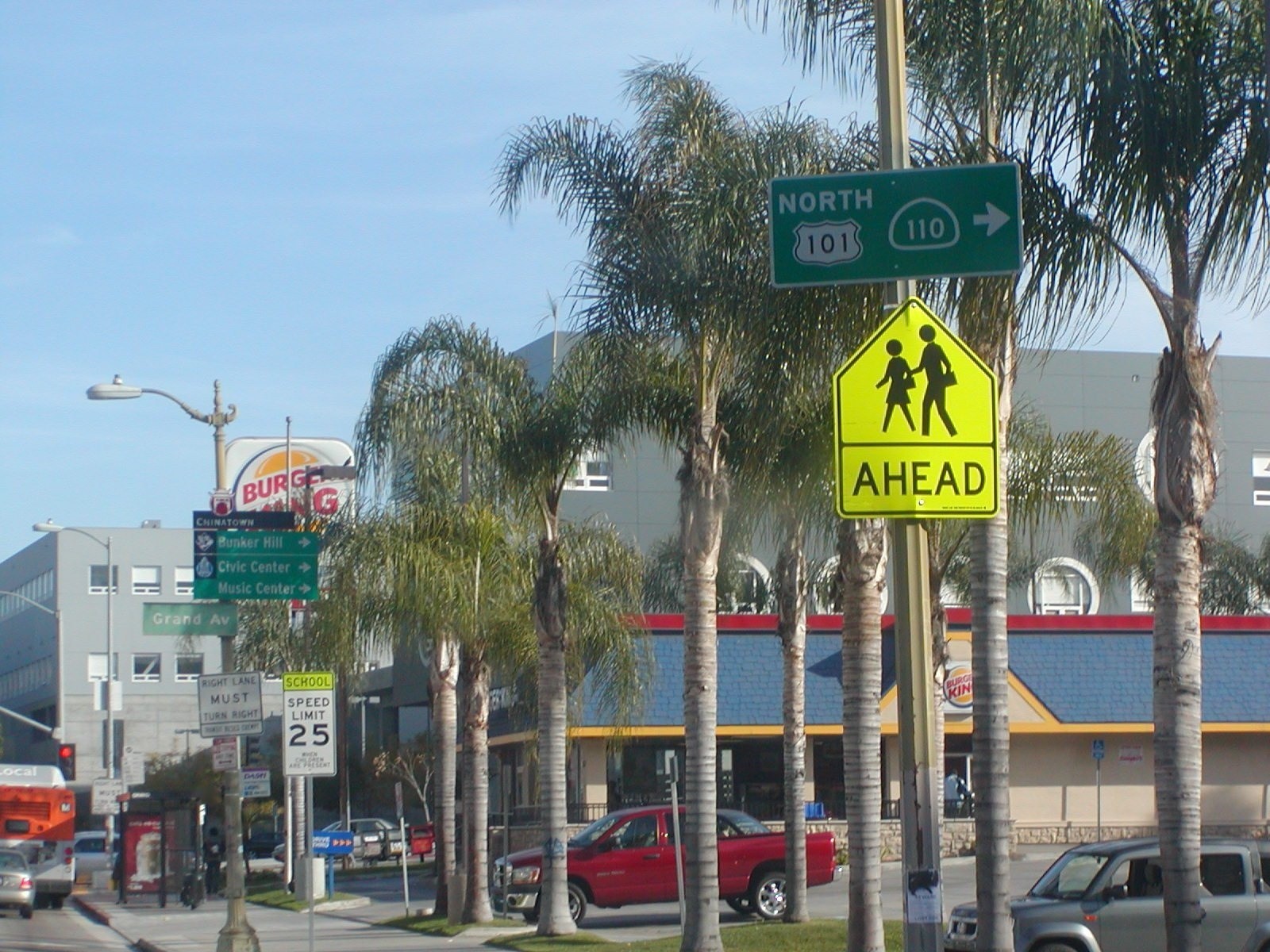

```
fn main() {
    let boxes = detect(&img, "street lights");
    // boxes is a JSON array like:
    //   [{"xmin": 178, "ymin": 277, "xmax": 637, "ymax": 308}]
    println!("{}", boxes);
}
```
[
  {"xmin": 176, "ymin": 728, "xmax": 201, "ymax": 760},
  {"xmin": 32, "ymin": 519, "xmax": 114, "ymax": 857},
  {"xmin": 292, "ymin": 463, "xmax": 359, "ymax": 903},
  {"xmin": 349, "ymin": 694, "xmax": 382, "ymax": 815},
  {"xmin": 86, "ymin": 375, "xmax": 261, "ymax": 952}
]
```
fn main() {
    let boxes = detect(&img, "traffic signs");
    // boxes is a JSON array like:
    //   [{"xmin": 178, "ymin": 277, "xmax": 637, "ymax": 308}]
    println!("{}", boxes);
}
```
[
  {"xmin": 211, "ymin": 737, "xmax": 239, "ymax": 773},
  {"xmin": 766, "ymin": 165, "xmax": 1024, "ymax": 288}
]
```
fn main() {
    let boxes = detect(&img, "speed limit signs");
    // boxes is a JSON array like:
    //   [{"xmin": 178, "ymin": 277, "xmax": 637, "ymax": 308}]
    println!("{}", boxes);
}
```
[{"xmin": 283, "ymin": 689, "xmax": 338, "ymax": 778}]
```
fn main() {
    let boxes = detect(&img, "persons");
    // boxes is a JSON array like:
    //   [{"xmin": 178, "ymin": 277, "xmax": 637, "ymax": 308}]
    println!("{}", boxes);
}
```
[
  {"xmin": 944, "ymin": 768, "xmax": 971, "ymax": 817},
  {"xmin": 204, "ymin": 828, "xmax": 223, "ymax": 896}
]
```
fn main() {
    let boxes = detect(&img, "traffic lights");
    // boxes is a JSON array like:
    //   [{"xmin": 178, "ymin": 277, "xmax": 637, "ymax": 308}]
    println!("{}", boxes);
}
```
[
  {"xmin": 657, "ymin": 773, "xmax": 675, "ymax": 800},
  {"xmin": 245, "ymin": 736, "xmax": 261, "ymax": 766},
  {"xmin": 60, "ymin": 742, "xmax": 78, "ymax": 782}
]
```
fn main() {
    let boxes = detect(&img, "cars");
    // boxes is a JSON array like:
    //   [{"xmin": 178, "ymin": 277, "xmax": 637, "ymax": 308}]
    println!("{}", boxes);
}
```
[
  {"xmin": 1, "ymin": 848, "xmax": 36, "ymax": 919},
  {"xmin": 73, "ymin": 831, "xmax": 122, "ymax": 885},
  {"xmin": 270, "ymin": 816, "xmax": 410, "ymax": 866},
  {"xmin": 945, "ymin": 836, "xmax": 1268, "ymax": 952},
  {"xmin": 491, "ymin": 801, "xmax": 841, "ymax": 925}
]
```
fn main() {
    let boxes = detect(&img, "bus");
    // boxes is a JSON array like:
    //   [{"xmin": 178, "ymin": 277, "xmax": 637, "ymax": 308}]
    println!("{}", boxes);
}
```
[{"xmin": 0, "ymin": 763, "xmax": 77, "ymax": 909}]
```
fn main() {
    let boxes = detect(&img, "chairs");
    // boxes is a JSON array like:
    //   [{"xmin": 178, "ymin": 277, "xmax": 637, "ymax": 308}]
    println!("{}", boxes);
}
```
[{"xmin": 1136, "ymin": 863, "xmax": 1163, "ymax": 897}]
